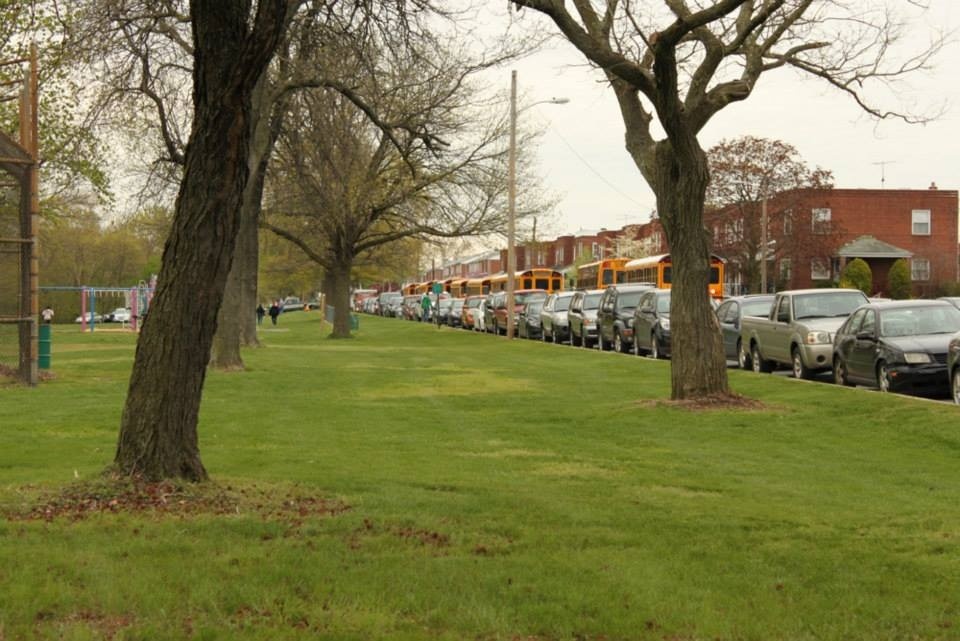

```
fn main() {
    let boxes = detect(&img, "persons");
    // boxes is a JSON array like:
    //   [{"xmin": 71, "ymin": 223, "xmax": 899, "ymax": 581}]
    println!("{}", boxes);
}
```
[
  {"xmin": 268, "ymin": 300, "xmax": 280, "ymax": 325},
  {"xmin": 42, "ymin": 306, "xmax": 55, "ymax": 324},
  {"xmin": 421, "ymin": 292, "xmax": 433, "ymax": 322},
  {"xmin": 256, "ymin": 304, "xmax": 265, "ymax": 325}
]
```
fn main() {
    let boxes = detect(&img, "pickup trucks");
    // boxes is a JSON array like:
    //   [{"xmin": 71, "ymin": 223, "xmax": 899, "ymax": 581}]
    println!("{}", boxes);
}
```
[{"xmin": 740, "ymin": 288, "xmax": 871, "ymax": 379}]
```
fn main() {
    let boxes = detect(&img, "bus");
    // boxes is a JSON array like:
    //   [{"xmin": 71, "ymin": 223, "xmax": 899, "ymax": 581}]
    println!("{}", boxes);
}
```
[
  {"xmin": 489, "ymin": 268, "xmax": 564, "ymax": 296},
  {"xmin": 624, "ymin": 254, "xmax": 728, "ymax": 304},
  {"xmin": 569, "ymin": 257, "xmax": 632, "ymax": 290},
  {"xmin": 403, "ymin": 280, "xmax": 467, "ymax": 298},
  {"xmin": 464, "ymin": 277, "xmax": 490, "ymax": 297}
]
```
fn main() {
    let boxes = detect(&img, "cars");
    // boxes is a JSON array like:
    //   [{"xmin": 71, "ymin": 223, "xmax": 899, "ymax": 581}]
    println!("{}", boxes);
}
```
[
  {"xmin": 715, "ymin": 294, "xmax": 776, "ymax": 370},
  {"xmin": 540, "ymin": 291, "xmax": 579, "ymax": 344},
  {"xmin": 595, "ymin": 284, "xmax": 655, "ymax": 354},
  {"xmin": 949, "ymin": 331, "xmax": 960, "ymax": 404},
  {"xmin": 567, "ymin": 290, "xmax": 606, "ymax": 348},
  {"xmin": 349, "ymin": 289, "xmax": 486, "ymax": 331},
  {"xmin": 472, "ymin": 301, "xmax": 487, "ymax": 332},
  {"xmin": 832, "ymin": 297, "xmax": 960, "ymax": 393},
  {"xmin": 484, "ymin": 291, "xmax": 505, "ymax": 333},
  {"xmin": 75, "ymin": 307, "xmax": 131, "ymax": 323},
  {"xmin": 633, "ymin": 289, "xmax": 719, "ymax": 360},
  {"xmin": 494, "ymin": 289, "xmax": 545, "ymax": 337},
  {"xmin": 938, "ymin": 297, "xmax": 960, "ymax": 309},
  {"xmin": 517, "ymin": 300, "xmax": 543, "ymax": 340}
]
